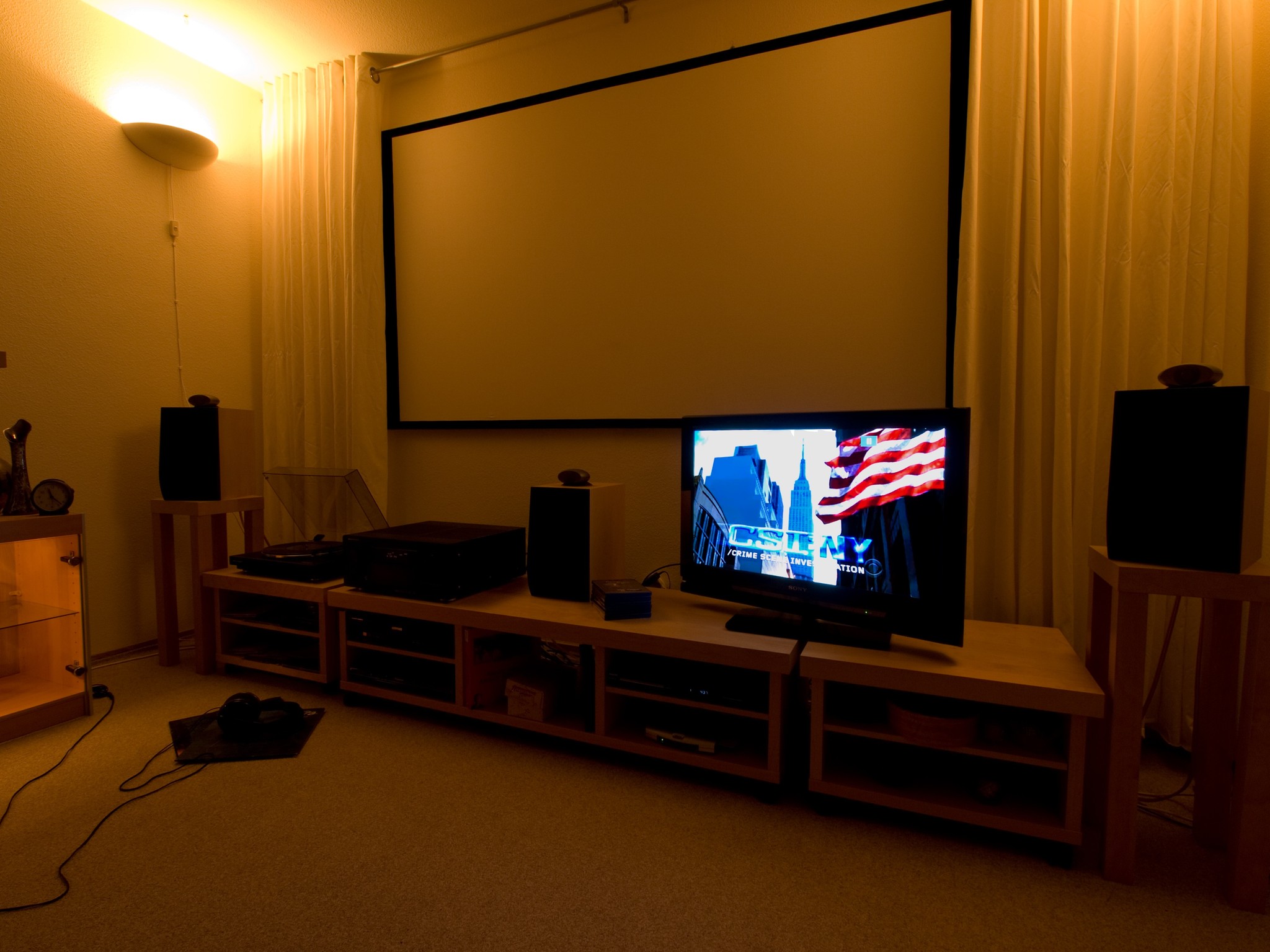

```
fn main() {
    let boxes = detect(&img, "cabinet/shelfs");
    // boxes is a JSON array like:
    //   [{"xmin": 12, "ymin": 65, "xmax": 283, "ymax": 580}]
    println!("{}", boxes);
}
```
[
  {"xmin": 198, "ymin": 551, "xmax": 1107, "ymax": 867},
  {"xmin": 1, "ymin": 512, "xmax": 98, "ymax": 748}
]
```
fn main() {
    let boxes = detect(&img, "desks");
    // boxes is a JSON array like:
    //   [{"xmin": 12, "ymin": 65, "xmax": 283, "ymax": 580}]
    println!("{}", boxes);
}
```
[
  {"xmin": 146, "ymin": 494, "xmax": 265, "ymax": 680},
  {"xmin": 1083, "ymin": 541, "xmax": 1270, "ymax": 913}
]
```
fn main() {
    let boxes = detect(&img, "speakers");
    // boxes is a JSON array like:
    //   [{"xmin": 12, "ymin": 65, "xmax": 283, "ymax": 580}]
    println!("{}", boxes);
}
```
[
  {"xmin": 527, "ymin": 479, "xmax": 626, "ymax": 601},
  {"xmin": 158, "ymin": 407, "xmax": 257, "ymax": 500},
  {"xmin": 1106, "ymin": 387, "xmax": 1269, "ymax": 571}
]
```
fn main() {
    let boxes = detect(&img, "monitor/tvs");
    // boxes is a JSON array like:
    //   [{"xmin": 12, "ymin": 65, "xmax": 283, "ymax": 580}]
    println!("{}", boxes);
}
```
[{"xmin": 679, "ymin": 409, "xmax": 971, "ymax": 647}]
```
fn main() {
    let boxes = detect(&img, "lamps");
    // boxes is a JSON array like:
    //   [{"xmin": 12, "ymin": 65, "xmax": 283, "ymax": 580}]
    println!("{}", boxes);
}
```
[{"xmin": 121, "ymin": 121, "xmax": 219, "ymax": 171}]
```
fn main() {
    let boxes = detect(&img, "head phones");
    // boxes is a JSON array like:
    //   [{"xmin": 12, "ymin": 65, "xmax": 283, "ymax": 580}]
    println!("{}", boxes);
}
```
[{"xmin": 217, "ymin": 691, "xmax": 305, "ymax": 733}]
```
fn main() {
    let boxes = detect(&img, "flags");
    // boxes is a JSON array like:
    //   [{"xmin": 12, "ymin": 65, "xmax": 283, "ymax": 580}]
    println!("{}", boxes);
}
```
[{"xmin": 817, "ymin": 427, "xmax": 946, "ymax": 525}]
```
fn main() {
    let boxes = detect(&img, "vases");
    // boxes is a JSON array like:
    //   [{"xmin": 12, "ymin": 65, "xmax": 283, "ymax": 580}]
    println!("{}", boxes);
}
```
[{"xmin": 2, "ymin": 418, "xmax": 36, "ymax": 516}]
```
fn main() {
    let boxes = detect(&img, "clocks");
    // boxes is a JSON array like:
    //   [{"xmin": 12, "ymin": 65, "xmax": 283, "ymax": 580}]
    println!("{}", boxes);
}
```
[{"xmin": 30, "ymin": 478, "xmax": 75, "ymax": 517}]
